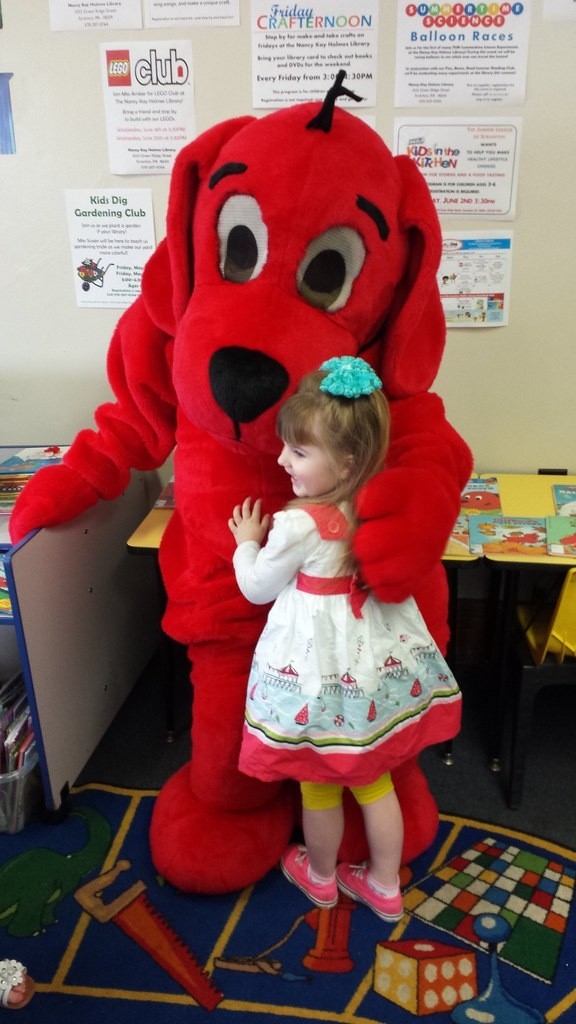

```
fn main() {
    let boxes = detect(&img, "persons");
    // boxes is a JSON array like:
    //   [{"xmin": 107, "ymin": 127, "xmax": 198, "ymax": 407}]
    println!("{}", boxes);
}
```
[{"xmin": 227, "ymin": 355, "xmax": 465, "ymax": 922}]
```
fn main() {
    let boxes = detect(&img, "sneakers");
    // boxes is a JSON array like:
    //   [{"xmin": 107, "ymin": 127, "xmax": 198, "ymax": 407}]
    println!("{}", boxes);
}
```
[
  {"xmin": 281, "ymin": 839, "xmax": 338, "ymax": 909},
  {"xmin": 335, "ymin": 862, "xmax": 404, "ymax": 923}
]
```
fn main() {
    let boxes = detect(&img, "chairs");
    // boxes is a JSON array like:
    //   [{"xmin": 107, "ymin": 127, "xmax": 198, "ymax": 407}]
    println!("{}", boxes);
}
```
[{"xmin": 504, "ymin": 566, "xmax": 575, "ymax": 809}]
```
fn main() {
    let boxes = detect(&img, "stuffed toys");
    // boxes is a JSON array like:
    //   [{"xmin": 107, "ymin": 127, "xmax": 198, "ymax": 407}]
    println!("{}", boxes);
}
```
[{"xmin": 11, "ymin": 70, "xmax": 473, "ymax": 890}]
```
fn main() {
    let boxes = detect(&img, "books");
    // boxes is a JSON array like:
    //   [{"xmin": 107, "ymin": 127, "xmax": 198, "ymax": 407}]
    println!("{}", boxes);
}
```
[{"xmin": 451, "ymin": 476, "xmax": 575, "ymax": 560}]
